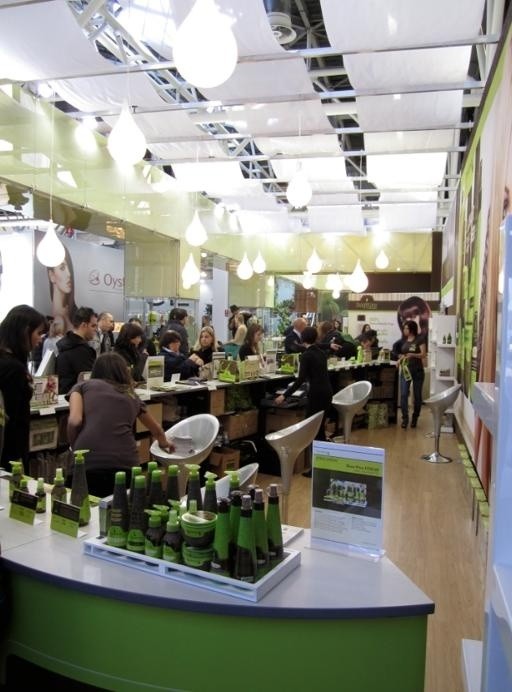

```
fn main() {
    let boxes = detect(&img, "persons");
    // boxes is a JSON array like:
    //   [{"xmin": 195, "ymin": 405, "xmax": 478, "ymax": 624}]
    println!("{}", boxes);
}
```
[
  {"xmin": 0, "ymin": 305, "xmax": 50, "ymax": 474},
  {"xmin": 283, "ymin": 319, "xmax": 295, "ymax": 336},
  {"xmin": 88, "ymin": 312, "xmax": 114, "ymax": 360},
  {"xmin": 315, "ymin": 322, "xmax": 358, "ymax": 361},
  {"xmin": 272, "ymin": 327, "xmax": 339, "ymax": 478},
  {"xmin": 128, "ymin": 318, "xmax": 157, "ymax": 356},
  {"xmin": 42, "ymin": 307, "xmax": 98, "ymax": 395},
  {"xmin": 285, "ymin": 318, "xmax": 343, "ymax": 354},
  {"xmin": 158, "ymin": 330, "xmax": 203, "ymax": 382},
  {"xmin": 66, "ymin": 351, "xmax": 177, "ymax": 497},
  {"xmin": 331, "ymin": 320, "xmax": 342, "ymax": 331},
  {"xmin": 201, "ymin": 315, "xmax": 214, "ymax": 328},
  {"xmin": 28, "ymin": 314, "xmax": 54, "ymax": 377},
  {"xmin": 217, "ymin": 315, "xmax": 247, "ymax": 361},
  {"xmin": 390, "ymin": 296, "xmax": 433, "ymax": 368},
  {"xmin": 189, "ymin": 327, "xmax": 225, "ymax": 365},
  {"xmin": 237, "ymin": 323, "xmax": 267, "ymax": 371},
  {"xmin": 116, "ymin": 323, "xmax": 150, "ymax": 381},
  {"xmin": 361, "ymin": 324, "xmax": 371, "ymax": 334},
  {"xmin": 47, "ymin": 244, "xmax": 79, "ymax": 335},
  {"xmin": 158, "ymin": 308, "xmax": 190, "ymax": 357},
  {"xmin": 228, "ymin": 304, "xmax": 260, "ymax": 339},
  {"xmin": 390, "ymin": 321, "xmax": 426, "ymax": 429}
]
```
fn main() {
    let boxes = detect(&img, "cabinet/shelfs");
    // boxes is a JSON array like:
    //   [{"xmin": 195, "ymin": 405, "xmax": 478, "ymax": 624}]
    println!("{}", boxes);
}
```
[{"xmin": 433, "ymin": 315, "xmax": 456, "ymax": 434}]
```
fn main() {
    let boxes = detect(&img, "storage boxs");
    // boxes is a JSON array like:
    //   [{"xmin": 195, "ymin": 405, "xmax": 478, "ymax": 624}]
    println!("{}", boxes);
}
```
[
  {"xmin": 136, "ymin": 437, "xmax": 151, "ymax": 465},
  {"xmin": 266, "ymin": 402, "xmax": 298, "ymax": 433},
  {"xmin": 211, "ymin": 448, "xmax": 240, "ymax": 475},
  {"xmin": 221, "ymin": 408, "xmax": 258, "ymax": 440},
  {"xmin": 200, "ymin": 390, "xmax": 225, "ymax": 416},
  {"xmin": 136, "ymin": 401, "xmax": 163, "ymax": 434}
]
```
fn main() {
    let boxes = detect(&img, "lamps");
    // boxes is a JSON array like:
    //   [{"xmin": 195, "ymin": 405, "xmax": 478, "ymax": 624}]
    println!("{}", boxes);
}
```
[
  {"xmin": 107, "ymin": 106, "xmax": 148, "ymax": 169},
  {"xmin": 305, "ymin": 253, "xmax": 323, "ymax": 274},
  {"xmin": 302, "ymin": 270, "xmax": 314, "ymax": 289},
  {"xmin": 237, "ymin": 247, "xmax": 254, "ymax": 280},
  {"xmin": 35, "ymin": 217, "xmax": 65, "ymax": 268},
  {"xmin": 347, "ymin": 150, "xmax": 369, "ymax": 294},
  {"xmin": 374, "ymin": 249, "xmax": 389, "ymax": 269},
  {"xmin": 286, "ymin": 92, "xmax": 313, "ymax": 211},
  {"xmin": 172, "ymin": 0, "xmax": 238, "ymax": 90},
  {"xmin": 181, "ymin": 254, "xmax": 201, "ymax": 285},
  {"xmin": 251, "ymin": 246, "xmax": 266, "ymax": 275},
  {"xmin": 184, "ymin": 135, "xmax": 208, "ymax": 248}
]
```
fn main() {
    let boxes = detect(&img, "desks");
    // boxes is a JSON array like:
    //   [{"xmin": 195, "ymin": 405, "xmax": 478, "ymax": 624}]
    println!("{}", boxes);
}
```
[
  {"xmin": 0, "ymin": 469, "xmax": 102, "ymax": 553},
  {"xmin": 135, "ymin": 385, "xmax": 209, "ymax": 398},
  {"xmin": 177, "ymin": 373, "xmax": 299, "ymax": 391},
  {"xmin": 325, "ymin": 359, "xmax": 399, "ymax": 425},
  {"xmin": 29, "ymin": 393, "xmax": 71, "ymax": 415},
  {"xmin": 0, "ymin": 518, "xmax": 435, "ymax": 692}
]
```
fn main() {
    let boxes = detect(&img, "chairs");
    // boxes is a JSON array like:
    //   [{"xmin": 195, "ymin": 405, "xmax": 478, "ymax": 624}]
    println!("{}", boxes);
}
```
[
  {"xmin": 179, "ymin": 463, "xmax": 259, "ymax": 510},
  {"xmin": 330, "ymin": 381, "xmax": 373, "ymax": 443},
  {"xmin": 422, "ymin": 381, "xmax": 461, "ymax": 464},
  {"xmin": 260, "ymin": 409, "xmax": 327, "ymax": 525}
]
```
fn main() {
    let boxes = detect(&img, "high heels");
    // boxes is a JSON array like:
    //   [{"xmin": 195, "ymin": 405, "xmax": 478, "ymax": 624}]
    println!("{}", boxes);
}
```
[
  {"xmin": 410, "ymin": 421, "xmax": 417, "ymax": 427},
  {"xmin": 401, "ymin": 422, "xmax": 407, "ymax": 429}
]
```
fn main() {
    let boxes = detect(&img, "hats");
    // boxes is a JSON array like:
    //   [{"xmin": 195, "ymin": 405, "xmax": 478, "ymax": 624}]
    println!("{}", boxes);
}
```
[{"xmin": 229, "ymin": 304, "xmax": 239, "ymax": 312}]
